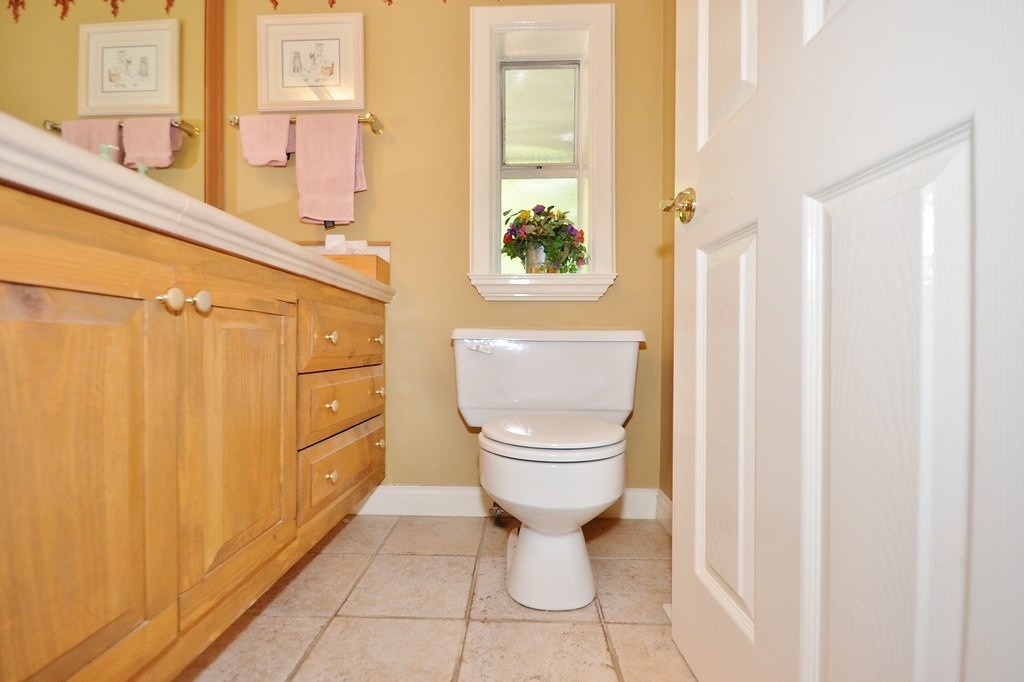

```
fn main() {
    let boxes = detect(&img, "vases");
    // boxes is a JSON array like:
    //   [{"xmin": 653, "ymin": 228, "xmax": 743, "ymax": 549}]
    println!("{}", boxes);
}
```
[{"xmin": 525, "ymin": 238, "xmax": 558, "ymax": 274}]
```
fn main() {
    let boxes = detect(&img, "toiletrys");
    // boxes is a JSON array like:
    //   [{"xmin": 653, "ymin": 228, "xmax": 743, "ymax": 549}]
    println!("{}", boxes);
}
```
[
  {"xmin": 98, "ymin": 144, "xmax": 119, "ymax": 161},
  {"xmin": 133, "ymin": 160, "xmax": 148, "ymax": 175}
]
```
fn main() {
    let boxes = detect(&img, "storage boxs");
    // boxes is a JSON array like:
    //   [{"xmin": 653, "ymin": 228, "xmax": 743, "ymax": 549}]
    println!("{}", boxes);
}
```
[{"xmin": 294, "ymin": 241, "xmax": 390, "ymax": 285}]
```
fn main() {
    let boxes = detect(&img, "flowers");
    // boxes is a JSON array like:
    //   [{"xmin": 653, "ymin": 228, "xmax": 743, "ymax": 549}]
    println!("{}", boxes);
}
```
[{"xmin": 500, "ymin": 205, "xmax": 591, "ymax": 274}]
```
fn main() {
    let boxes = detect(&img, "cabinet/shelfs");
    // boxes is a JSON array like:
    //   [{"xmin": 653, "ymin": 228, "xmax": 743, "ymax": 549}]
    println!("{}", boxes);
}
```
[{"xmin": 0, "ymin": 180, "xmax": 385, "ymax": 682}]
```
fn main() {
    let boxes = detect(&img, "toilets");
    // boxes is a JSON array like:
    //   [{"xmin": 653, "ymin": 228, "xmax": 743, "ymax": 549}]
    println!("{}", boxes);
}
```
[{"xmin": 445, "ymin": 327, "xmax": 646, "ymax": 609}]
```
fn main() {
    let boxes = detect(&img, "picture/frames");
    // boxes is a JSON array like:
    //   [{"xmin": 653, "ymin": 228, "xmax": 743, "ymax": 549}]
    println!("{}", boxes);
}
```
[
  {"xmin": 76, "ymin": 20, "xmax": 180, "ymax": 117},
  {"xmin": 256, "ymin": 12, "xmax": 366, "ymax": 113}
]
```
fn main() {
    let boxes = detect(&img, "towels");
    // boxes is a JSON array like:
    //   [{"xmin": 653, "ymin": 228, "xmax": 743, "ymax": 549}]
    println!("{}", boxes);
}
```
[
  {"xmin": 59, "ymin": 120, "xmax": 122, "ymax": 165},
  {"xmin": 123, "ymin": 117, "xmax": 184, "ymax": 169},
  {"xmin": 297, "ymin": 116, "xmax": 369, "ymax": 230},
  {"xmin": 236, "ymin": 115, "xmax": 296, "ymax": 169}
]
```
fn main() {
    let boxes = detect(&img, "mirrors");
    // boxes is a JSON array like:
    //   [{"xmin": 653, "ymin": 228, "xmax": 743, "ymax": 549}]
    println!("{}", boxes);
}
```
[{"xmin": 0, "ymin": 0, "xmax": 205, "ymax": 203}]
